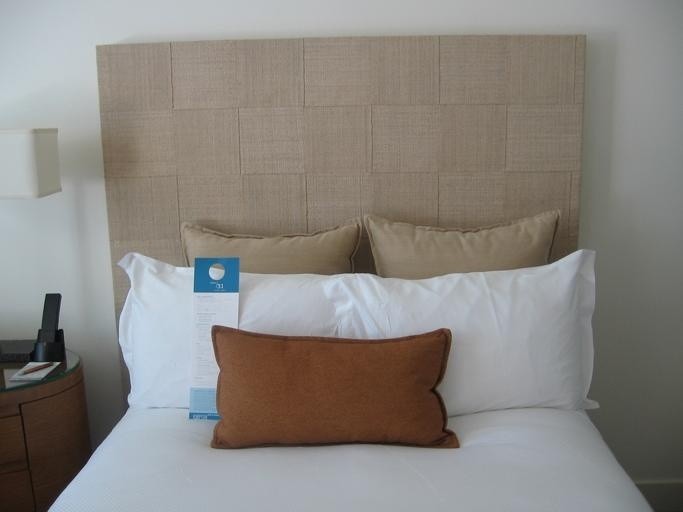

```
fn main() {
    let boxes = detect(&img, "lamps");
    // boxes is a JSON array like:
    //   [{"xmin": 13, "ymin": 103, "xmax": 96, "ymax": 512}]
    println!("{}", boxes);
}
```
[{"xmin": 1, "ymin": 127, "xmax": 62, "ymax": 198}]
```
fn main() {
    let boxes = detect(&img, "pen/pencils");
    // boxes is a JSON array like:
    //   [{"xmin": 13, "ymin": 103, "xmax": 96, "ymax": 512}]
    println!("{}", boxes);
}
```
[{"xmin": 23, "ymin": 362, "xmax": 54, "ymax": 374}]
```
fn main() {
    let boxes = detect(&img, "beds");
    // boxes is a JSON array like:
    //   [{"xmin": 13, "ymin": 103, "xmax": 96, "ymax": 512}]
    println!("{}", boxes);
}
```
[{"xmin": 47, "ymin": 407, "xmax": 654, "ymax": 512}]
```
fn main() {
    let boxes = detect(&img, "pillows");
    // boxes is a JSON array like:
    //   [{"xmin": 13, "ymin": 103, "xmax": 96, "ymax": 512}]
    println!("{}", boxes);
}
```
[
  {"xmin": 362, "ymin": 208, "xmax": 562, "ymax": 280},
  {"xmin": 320, "ymin": 248, "xmax": 600, "ymax": 417},
  {"xmin": 180, "ymin": 216, "xmax": 363, "ymax": 275},
  {"xmin": 116, "ymin": 251, "xmax": 382, "ymax": 411},
  {"xmin": 210, "ymin": 325, "xmax": 460, "ymax": 449}
]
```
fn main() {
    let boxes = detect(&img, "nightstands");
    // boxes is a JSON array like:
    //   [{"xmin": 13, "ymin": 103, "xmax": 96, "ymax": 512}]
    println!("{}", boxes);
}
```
[{"xmin": 0, "ymin": 349, "xmax": 91, "ymax": 512}]
